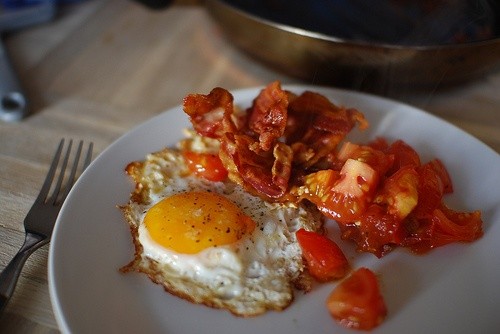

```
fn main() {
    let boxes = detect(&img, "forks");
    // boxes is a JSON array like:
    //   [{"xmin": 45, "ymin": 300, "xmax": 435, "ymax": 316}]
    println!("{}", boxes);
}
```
[{"xmin": 0, "ymin": 136, "xmax": 94, "ymax": 317}]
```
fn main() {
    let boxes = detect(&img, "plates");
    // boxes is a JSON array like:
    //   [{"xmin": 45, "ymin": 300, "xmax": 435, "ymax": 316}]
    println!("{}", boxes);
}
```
[{"xmin": 46, "ymin": 84, "xmax": 499, "ymax": 333}]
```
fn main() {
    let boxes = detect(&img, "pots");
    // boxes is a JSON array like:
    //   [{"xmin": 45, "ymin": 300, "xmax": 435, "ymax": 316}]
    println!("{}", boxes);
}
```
[{"xmin": 132, "ymin": 0, "xmax": 500, "ymax": 96}]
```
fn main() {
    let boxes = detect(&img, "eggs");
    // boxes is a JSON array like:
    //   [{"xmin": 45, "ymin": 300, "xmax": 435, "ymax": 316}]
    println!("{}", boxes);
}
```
[{"xmin": 119, "ymin": 147, "xmax": 327, "ymax": 320}]
